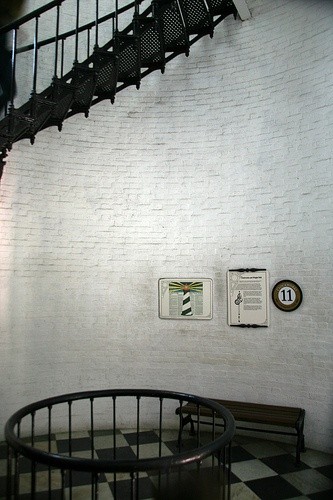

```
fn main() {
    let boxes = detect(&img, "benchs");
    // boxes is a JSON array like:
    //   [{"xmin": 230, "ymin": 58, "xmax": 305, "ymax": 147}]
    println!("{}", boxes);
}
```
[{"xmin": 175, "ymin": 398, "xmax": 305, "ymax": 466}]
